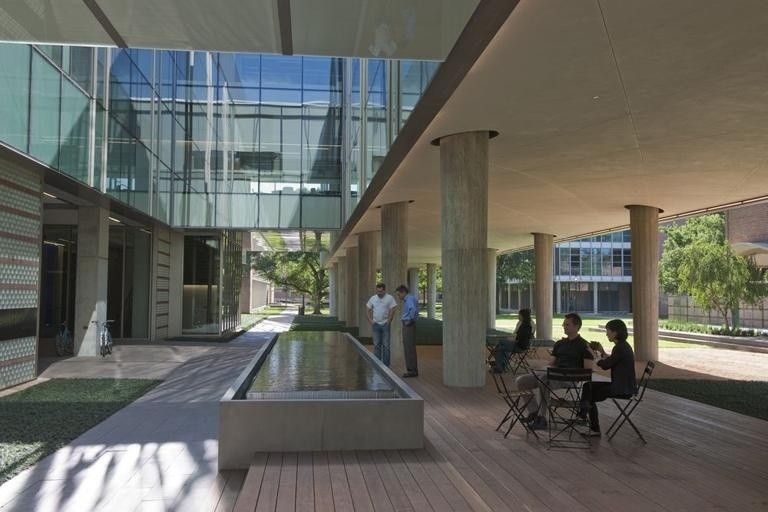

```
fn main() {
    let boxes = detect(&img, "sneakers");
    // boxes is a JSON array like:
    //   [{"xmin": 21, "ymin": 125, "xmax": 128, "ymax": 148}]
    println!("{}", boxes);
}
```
[
  {"xmin": 403, "ymin": 368, "xmax": 418, "ymax": 377},
  {"xmin": 581, "ymin": 427, "xmax": 600, "ymax": 436},
  {"xmin": 520, "ymin": 411, "xmax": 547, "ymax": 429},
  {"xmin": 488, "ymin": 361, "xmax": 503, "ymax": 374},
  {"xmin": 567, "ymin": 416, "xmax": 585, "ymax": 425}
]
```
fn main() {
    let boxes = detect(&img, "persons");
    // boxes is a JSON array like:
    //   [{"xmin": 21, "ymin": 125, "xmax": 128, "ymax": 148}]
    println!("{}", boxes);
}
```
[
  {"xmin": 365, "ymin": 283, "xmax": 398, "ymax": 366},
  {"xmin": 567, "ymin": 318, "xmax": 638, "ymax": 436},
  {"xmin": 488, "ymin": 309, "xmax": 532, "ymax": 373},
  {"xmin": 395, "ymin": 284, "xmax": 420, "ymax": 378},
  {"xmin": 512, "ymin": 309, "xmax": 536, "ymax": 338},
  {"xmin": 514, "ymin": 312, "xmax": 597, "ymax": 430}
]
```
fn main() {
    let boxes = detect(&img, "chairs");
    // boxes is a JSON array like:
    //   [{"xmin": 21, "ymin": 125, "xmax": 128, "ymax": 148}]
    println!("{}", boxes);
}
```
[{"xmin": 486, "ymin": 331, "xmax": 656, "ymax": 450}]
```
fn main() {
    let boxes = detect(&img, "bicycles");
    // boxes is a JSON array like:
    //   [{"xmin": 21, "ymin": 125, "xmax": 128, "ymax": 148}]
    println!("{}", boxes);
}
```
[
  {"xmin": 90, "ymin": 319, "xmax": 115, "ymax": 358},
  {"xmin": 53, "ymin": 318, "xmax": 72, "ymax": 356}
]
